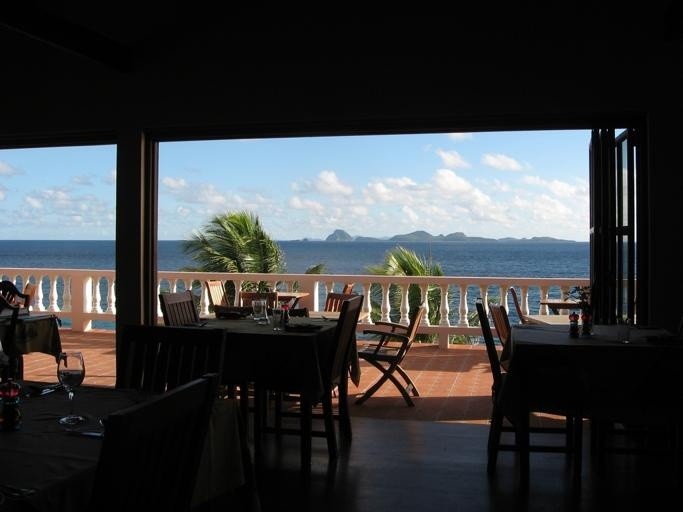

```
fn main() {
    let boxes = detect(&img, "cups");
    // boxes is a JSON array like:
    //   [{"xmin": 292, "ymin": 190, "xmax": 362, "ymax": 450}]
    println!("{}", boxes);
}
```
[
  {"xmin": 617, "ymin": 319, "xmax": 632, "ymax": 343},
  {"xmin": 252, "ymin": 299, "xmax": 267, "ymax": 322}
]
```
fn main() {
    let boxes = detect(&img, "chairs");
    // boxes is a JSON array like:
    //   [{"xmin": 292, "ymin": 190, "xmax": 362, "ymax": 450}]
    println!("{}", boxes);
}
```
[
  {"xmin": 101, "ymin": 370, "xmax": 219, "ymax": 510},
  {"xmin": 479, "ymin": 288, "xmax": 586, "ymax": 482},
  {"xmin": 357, "ymin": 303, "xmax": 426, "ymax": 408},
  {"xmin": 134, "ymin": 281, "xmax": 364, "ymax": 475},
  {"xmin": 1, "ymin": 279, "xmax": 63, "ymax": 372}
]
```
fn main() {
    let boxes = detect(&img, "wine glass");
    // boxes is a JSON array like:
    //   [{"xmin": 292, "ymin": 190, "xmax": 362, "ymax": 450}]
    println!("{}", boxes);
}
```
[{"xmin": 54, "ymin": 351, "xmax": 87, "ymax": 425}]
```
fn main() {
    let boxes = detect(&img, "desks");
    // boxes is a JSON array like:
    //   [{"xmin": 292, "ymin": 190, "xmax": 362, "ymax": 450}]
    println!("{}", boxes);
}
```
[
  {"xmin": 498, "ymin": 325, "xmax": 683, "ymax": 499},
  {"xmin": 3, "ymin": 382, "xmax": 239, "ymax": 510}
]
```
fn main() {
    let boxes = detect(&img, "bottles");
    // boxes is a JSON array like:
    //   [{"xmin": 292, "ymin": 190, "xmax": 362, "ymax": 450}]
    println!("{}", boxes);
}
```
[
  {"xmin": 280, "ymin": 304, "xmax": 290, "ymax": 326},
  {"xmin": 0, "ymin": 379, "xmax": 24, "ymax": 431},
  {"xmin": 568, "ymin": 311, "xmax": 593, "ymax": 335}
]
